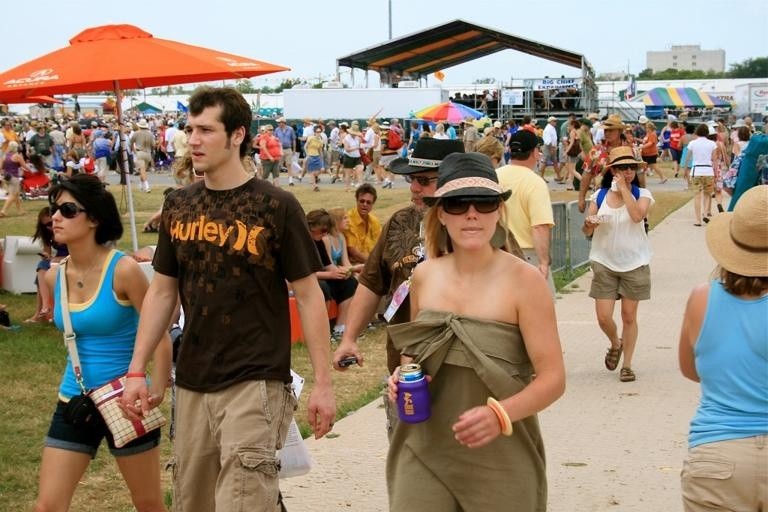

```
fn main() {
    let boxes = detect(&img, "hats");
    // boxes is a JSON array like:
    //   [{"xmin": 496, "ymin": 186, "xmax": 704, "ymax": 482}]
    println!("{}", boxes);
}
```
[
  {"xmin": 705, "ymin": 119, "xmax": 748, "ymax": 135},
  {"xmin": 275, "ymin": 117, "xmax": 389, "ymax": 135},
  {"xmin": 509, "ymin": 130, "xmax": 544, "ymax": 152},
  {"xmin": 705, "ymin": 184, "xmax": 767, "ymax": 277},
  {"xmin": 461, "ymin": 113, "xmax": 648, "ymax": 135},
  {"xmin": 422, "ymin": 152, "xmax": 511, "ymax": 207},
  {"xmin": 600, "ymin": 146, "xmax": 646, "ymax": 176},
  {"xmin": 135, "ymin": 119, "xmax": 148, "ymax": 128},
  {"xmin": 389, "ymin": 137, "xmax": 464, "ymax": 174}
]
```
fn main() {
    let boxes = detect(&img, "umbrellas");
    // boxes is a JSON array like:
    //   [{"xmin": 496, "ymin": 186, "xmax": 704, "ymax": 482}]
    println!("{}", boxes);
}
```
[
  {"xmin": 127, "ymin": 102, "xmax": 162, "ymax": 117},
  {"xmin": 415, "ymin": 101, "xmax": 485, "ymax": 125},
  {"xmin": 0, "ymin": 95, "xmax": 64, "ymax": 105},
  {"xmin": 1, "ymin": 25, "xmax": 292, "ymax": 252}
]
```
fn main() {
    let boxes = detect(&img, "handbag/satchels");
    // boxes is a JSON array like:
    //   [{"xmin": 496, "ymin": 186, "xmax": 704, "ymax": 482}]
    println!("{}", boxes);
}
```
[{"xmin": 85, "ymin": 375, "xmax": 166, "ymax": 448}]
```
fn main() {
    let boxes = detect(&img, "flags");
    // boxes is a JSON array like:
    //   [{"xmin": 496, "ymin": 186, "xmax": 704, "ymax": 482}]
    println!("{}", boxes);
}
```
[{"xmin": 177, "ymin": 101, "xmax": 188, "ymax": 113}]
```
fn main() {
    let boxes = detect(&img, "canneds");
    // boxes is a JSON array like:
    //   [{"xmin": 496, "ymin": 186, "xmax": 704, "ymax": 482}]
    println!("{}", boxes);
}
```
[{"xmin": 397, "ymin": 363, "xmax": 423, "ymax": 381}]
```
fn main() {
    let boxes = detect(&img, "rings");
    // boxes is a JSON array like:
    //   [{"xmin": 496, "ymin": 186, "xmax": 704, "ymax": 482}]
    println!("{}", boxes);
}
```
[{"xmin": 329, "ymin": 422, "xmax": 334, "ymax": 428}]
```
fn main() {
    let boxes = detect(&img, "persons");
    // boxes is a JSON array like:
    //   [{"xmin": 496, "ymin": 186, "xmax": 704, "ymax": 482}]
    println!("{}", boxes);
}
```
[
  {"xmin": 678, "ymin": 184, "xmax": 768, "ymax": 512},
  {"xmin": 246, "ymin": 114, "xmax": 450, "ymax": 191},
  {"xmin": 0, "ymin": 107, "xmax": 195, "ymax": 329},
  {"xmin": 445, "ymin": 84, "xmax": 580, "ymax": 116},
  {"xmin": 459, "ymin": 113, "xmax": 768, "ymax": 226},
  {"xmin": 495, "ymin": 129, "xmax": 555, "ymax": 296},
  {"xmin": 121, "ymin": 87, "xmax": 337, "ymax": 512},
  {"xmin": 582, "ymin": 146, "xmax": 655, "ymax": 385},
  {"xmin": 332, "ymin": 134, "xmax": 466, "ymax": 438},
  {"xmin": 33, "ymin": 173, "xmax": 173, "ymax": 512},
  {"xmin": 385, "ymin": 151, "xmax": 565, "ymax": 511},
  {"xmin": 301, "ymin": 203, "xmax": 378, "ymax": 341},
  {"xmin": 344, "ymin": 183, "xmax": 387, "ymax": 323}
]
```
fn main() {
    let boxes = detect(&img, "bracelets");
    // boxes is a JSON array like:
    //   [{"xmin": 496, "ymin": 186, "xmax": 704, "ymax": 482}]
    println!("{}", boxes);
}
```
[
  {"xmin": 486, "ymin": 394, "xmax": 514, "ymax": 439},
  {"xmin": 125, "ymin": 373, "xmax": 147, "ymax": 378}
]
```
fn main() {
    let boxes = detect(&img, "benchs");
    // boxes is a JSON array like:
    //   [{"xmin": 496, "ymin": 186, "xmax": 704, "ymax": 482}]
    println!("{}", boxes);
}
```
[
  {"xmin": 0, "ymin": 235, "xmax": 58, "ymax": 322},
  {"xmin": 288, "ymin": 296, "xmax": 339, "ymax": 345}
]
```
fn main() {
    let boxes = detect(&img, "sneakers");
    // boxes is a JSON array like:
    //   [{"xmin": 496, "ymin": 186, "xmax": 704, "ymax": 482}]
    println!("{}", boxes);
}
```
[{"xmin": 289, "ymin": 176, "xmax": 391, "ymax": 191}]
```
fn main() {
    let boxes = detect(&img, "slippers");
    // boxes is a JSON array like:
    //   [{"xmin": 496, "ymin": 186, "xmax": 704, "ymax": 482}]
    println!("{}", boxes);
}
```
[
  {"xmin": 619, "ymin": 369, "xmax": 634, "ymax": 382},
  {"xmin": 604, "ymin": 339, "xmax": 622, "ymax": 370}
]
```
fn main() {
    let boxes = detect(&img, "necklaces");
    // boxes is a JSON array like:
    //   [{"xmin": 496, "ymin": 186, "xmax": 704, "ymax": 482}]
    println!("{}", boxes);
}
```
[{"xmin": 68, "ymin": 259, "xmax": 96, "ymax": 288}]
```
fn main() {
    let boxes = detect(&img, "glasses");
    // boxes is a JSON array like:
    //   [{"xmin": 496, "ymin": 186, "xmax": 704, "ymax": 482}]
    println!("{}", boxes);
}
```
[
  {"xmin": 615, "ymin": 164, "xmax": 637, "ymax": 169},
  {"xmin": 403, "ymin": 174, "xmax": 436, "ymax": 186},
  {"xmin": 437, "ymin": 197, "xmax": 501, "ymax": 214},
  {"xmin": 360, "ymin": 199, "xmax": 371, "ymax": 204},
  {"xmin": 48, "ymin": 202, "xmax": 86, "ymax": 218}
]
